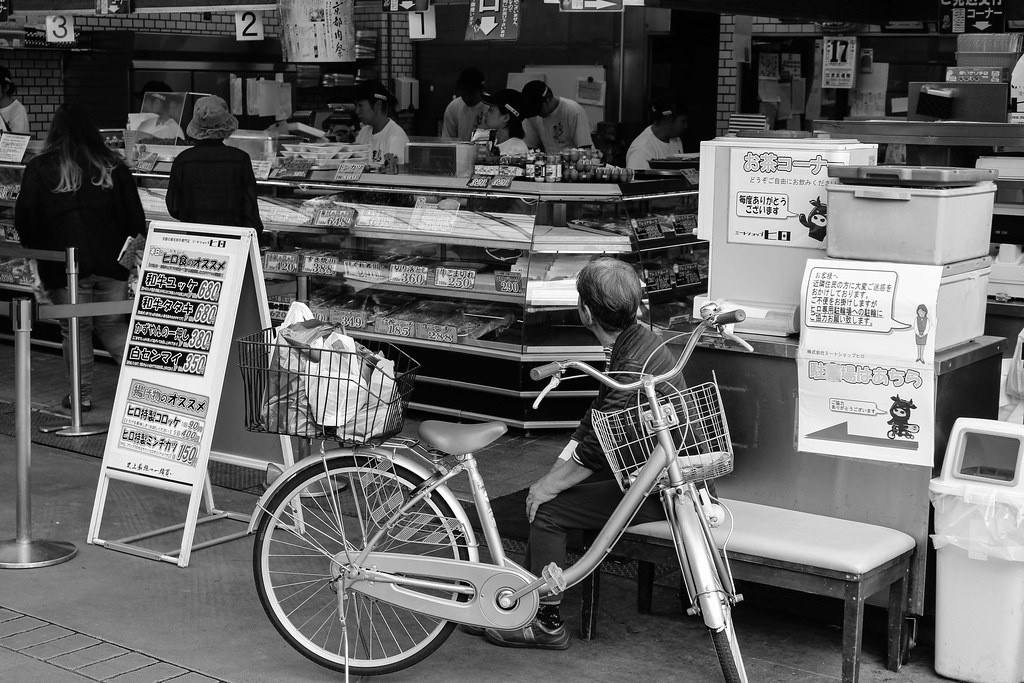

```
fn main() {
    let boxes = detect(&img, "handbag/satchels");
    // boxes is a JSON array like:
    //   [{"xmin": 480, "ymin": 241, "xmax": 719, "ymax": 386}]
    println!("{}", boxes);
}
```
[{"xmin": 259, "ymin": 301, "xmax": 402, "ymax": 441}]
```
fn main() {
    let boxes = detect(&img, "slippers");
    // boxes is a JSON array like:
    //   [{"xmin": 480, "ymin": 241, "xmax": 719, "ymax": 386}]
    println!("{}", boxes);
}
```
[{"xmin": 61, "ymin": 393, "xmax": 91, "ymax": 412}]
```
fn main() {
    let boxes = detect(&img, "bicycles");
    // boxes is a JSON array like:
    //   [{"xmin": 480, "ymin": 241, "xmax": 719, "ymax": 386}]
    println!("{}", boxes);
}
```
[{"xmin": 245, "ymin": 301, "xmax": 749, "ymax": 683}]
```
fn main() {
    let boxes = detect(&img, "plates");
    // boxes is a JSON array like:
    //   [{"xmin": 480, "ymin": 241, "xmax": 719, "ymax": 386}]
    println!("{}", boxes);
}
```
[{"xmin": 280, "ymin": 142, "xmax": 372, "ymax": 165}]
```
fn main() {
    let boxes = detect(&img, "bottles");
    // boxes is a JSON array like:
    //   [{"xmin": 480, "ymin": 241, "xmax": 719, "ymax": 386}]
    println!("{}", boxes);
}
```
[{"xmin": 499, "ymin": 147, "xmax": 562, "ymax": 182}]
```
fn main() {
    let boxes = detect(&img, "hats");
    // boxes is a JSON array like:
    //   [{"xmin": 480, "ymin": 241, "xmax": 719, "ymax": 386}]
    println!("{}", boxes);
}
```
[
  {"xmin": 458, "ymin": 78, "xmax": 485, "ymax": 107},
  {"xmin": 186, "ymin": 94, "xmax": 238, "ymax": 140},
  {"xmin": 0, "ymin": 65, "xmax": 13, "ymax": 81},
  {"xmin": 523, "ymin": 79, "xmax": 553, "ymax": 102},
  {"xmin": 481, "ymin": 89, "xmax": 527, "ymax": 122},
  {"xmin": 362, "ymin": 82, "xmax": 397, "ymax": 111},
  {"xmin": 650, "ymin": 98, "xmax": 686, "ymax": 123}
]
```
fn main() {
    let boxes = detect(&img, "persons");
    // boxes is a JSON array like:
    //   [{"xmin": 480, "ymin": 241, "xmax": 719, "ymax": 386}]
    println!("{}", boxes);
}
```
[
  {"xmin": 352, "ymin": 78, "xmax": 409, "ymax": 166},
  {"xmin": 0, "ymin": 65, "xmax": 29, "ymax": 133},
  {"xmin": 165, "ymin": 95, "xmax": 264, "ymax": 234},
  {"xmin": 476, "ymin": 89, "xmax": 528, "ymax": 153},
  {"xmin": 520, "ymin": 80, "xmax": 595, "ymax": 151},
  {"xmin": 16, "ymin": 102, "xmax": 146, "ymax": 410},
  {"xmin": 627, "ymin": 100, "xmax": 687, "ymax": 170},
  {"xmin": 463, "ymin": 256, "xmax": 702, "ymax": 649},
  {"xmin": 442, "ymin": 71, "xmax": 493, "ymax": 141},
  {"xmin": 133, "ymin": 79, "xmax": 176, "ymax": 124}
]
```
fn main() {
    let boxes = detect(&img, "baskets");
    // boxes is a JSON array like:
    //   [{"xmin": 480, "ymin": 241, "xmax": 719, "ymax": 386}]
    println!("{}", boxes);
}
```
[
  {"xmin": 234, "ymin": 327, "xmax": 421, "ymax": 446},
  {"xmin": 590, "ymin": 369, "xmax": 734, "ymax": 497}
]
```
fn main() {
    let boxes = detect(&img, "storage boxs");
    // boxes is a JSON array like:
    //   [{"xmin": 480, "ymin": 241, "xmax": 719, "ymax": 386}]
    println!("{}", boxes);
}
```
[
  {"xmin": 928, "ymin": 256, "xmax": 991, "ymax": 353},
  {"xmin": 826, "ymin": 165, "xmax": 999, "ymax": 266}
]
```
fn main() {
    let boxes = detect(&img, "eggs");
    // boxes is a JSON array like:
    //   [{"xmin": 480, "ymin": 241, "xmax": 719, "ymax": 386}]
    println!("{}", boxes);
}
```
[{"xmin": 560, "ymin": 147, "xmax": 633, "ymax": 182}]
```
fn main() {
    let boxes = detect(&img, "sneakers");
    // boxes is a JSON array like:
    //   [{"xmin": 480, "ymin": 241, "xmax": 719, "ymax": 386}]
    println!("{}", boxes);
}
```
[
  {"xmin": 483, "ymin": 619, "xmax": 569, "ymax": 650},
  {"xmin": 462, "ymin": 619, "xmax": 486, "ymax": 637}
]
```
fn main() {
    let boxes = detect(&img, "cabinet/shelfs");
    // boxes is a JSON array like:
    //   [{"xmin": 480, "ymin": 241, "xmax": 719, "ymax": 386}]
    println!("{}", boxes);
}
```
[{"xmin": 116, "ymin": 165, "xmax": 709, "ymax": 353}]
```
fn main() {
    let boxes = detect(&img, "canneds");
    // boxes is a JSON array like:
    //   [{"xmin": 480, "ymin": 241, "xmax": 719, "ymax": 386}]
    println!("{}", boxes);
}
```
[{"xmin": 534, "ymin": 154, "xmax": 562, "ymax": 183}]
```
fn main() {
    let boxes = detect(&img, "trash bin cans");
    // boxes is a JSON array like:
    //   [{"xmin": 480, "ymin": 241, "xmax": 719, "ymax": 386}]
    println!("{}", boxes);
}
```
[{"xmin": 930, "ymin": 417, "xmax": 1024, "ymax": 683}]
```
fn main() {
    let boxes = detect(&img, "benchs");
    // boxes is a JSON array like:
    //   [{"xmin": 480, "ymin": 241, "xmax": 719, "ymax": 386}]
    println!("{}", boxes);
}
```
[{"xmin": 583, "ymin": 498, "xmax": 916, "ymax": 683}]
